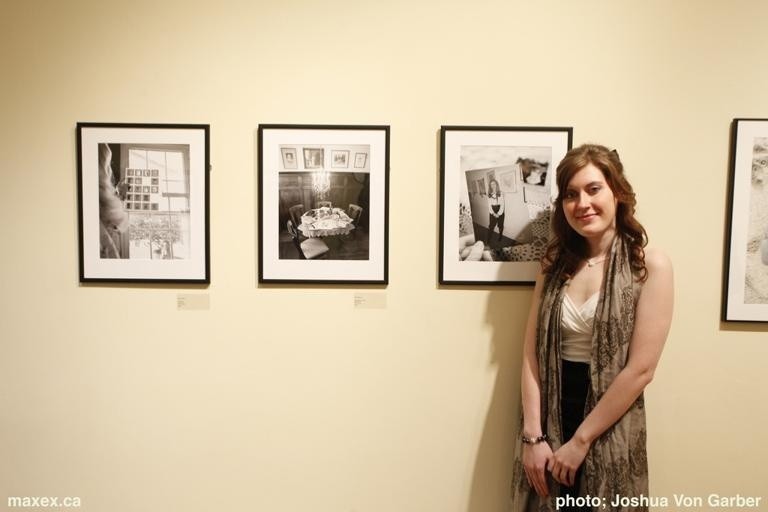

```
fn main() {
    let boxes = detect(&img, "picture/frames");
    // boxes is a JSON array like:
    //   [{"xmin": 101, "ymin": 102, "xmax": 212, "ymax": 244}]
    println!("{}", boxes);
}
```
[
  {"xmin": 437, "ymin": 123, "xmax": 574, "ymax": 286},
  {"xmin": 75, "ymin": 120, "xmax": 213, "ymax": 286},
  {"xmin": 720, "ymin": 117, "xmax": 768, "ymax": 326},
  {"xmin": 255, "ymin": 122, "xmax": 393, "ymax": 285}
]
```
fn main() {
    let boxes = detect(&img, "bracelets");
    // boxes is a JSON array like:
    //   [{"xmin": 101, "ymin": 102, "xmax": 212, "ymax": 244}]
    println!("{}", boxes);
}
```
[{"xmin": 520, "ymin": 432, "xmax": 548, "ymax": 446}]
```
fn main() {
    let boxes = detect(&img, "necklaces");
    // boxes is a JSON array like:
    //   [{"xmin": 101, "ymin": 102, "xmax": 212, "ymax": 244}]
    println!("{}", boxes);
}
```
[{"xmin": 580, "ymin": 253, "xmax": 609, "ymax": 269}]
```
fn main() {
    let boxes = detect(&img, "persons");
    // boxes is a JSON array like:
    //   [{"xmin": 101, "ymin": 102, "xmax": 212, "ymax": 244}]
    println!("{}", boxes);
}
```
[
  {"xmin": 484, "ymin": 177, "xmax": 506, "ymax": 245},
  {"xmin": 506, "ymin": 144, "xmax": 677, "ymax": 511}
]
